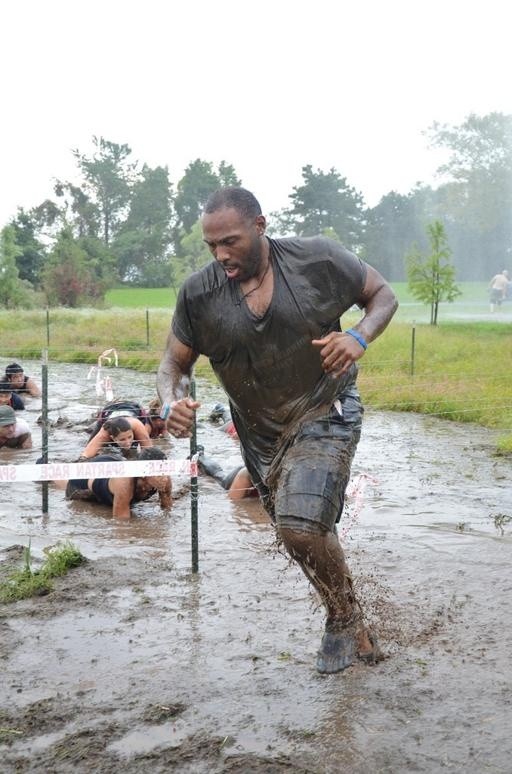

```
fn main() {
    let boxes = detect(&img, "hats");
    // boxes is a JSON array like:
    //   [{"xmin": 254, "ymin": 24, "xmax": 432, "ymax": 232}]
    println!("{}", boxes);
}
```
[{"xmin": 0, "ymin": 405, "xmax": 17, "ymax": 427}]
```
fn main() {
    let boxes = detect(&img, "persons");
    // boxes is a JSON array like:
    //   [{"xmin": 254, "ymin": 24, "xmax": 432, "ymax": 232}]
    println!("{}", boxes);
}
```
[
  {"xmin": 0, "ymin": 385, "xmax": 28, "ymax": 412},
  {"xmin": 487, "ymin": 270, "xmax": 509, "ymax": 314},
  {"xmin": 0, "ymin": 404, "xmax": 32, "ymax": 452},
  {"xmin": 154, "ymin": 186, "xmax": 400, "ymax": 674},
  {"xmin": 0, "ymin": 363, "xmax": 42, "ymax": 398},
  {"xmin": 34, "ymin": 389, "xmax": 275, "ymax": 519}
]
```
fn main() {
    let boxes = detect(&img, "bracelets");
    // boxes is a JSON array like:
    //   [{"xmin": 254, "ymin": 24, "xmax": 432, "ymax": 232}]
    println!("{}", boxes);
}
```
[{"xmin": 344, "ymin": 327, "xmax": 368, "ymax": 352}]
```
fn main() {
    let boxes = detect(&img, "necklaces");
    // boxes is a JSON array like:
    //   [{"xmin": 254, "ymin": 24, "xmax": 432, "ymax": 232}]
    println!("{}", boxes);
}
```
[{"xmin": 235, "ymin": 234, "xmax": 272, "ymax": 307}]
